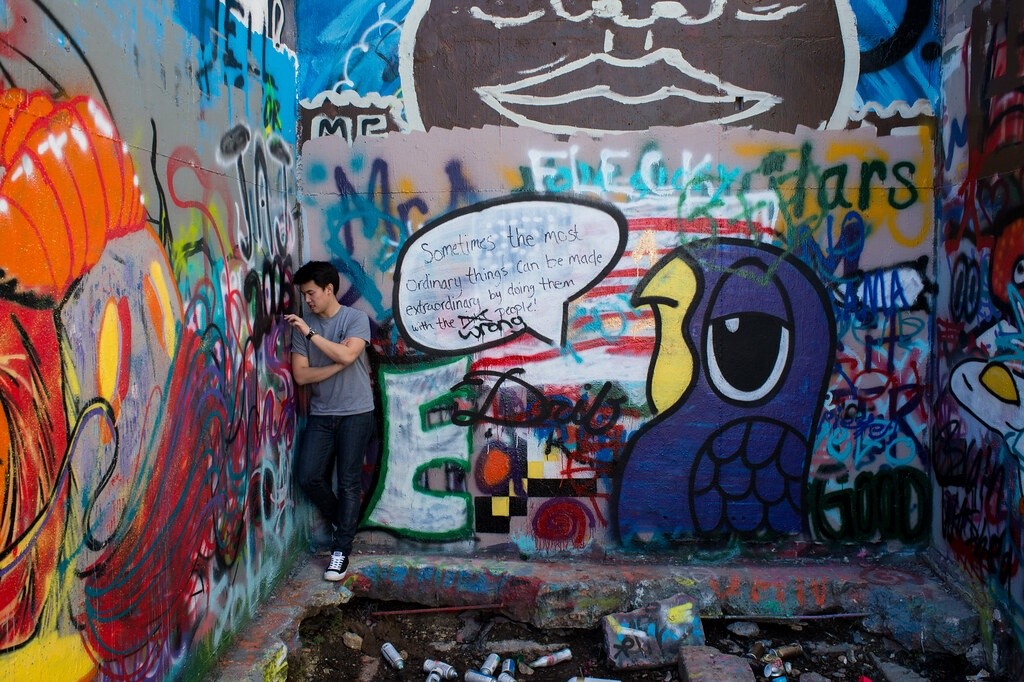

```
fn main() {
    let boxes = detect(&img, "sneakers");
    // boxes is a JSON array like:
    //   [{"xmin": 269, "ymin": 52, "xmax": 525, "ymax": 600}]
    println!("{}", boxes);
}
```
[
  {"xmin": 323, "ymin": 550, "xmax": 349, "ymax": 581},
  {"xmin": 330, "ymin": 522, "xmax": 337, "ymax": 545}
]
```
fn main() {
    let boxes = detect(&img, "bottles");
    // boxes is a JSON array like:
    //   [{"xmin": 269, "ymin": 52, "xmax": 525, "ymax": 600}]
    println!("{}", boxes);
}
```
[
  {"xmin": 465, "ymin": 653, "xmax": 518, "ymax": 682},
  {"xmin": 381, "ymin": 642, "xmax": 404, "ymax": 670},
  {"xmin": 529, "ymin": 648, "xmax": 572, "ymax": 667},
  {"xmin": 746, "ymin": 639, "xmax": 802, "ymax": 658},
  {"xmin": 423, "ymin": 658, "xmax": 459, "ymax": 682}
]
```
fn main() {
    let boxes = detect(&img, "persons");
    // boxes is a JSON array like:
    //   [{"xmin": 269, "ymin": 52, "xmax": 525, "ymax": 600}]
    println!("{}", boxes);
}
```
[{"xmin": 284, "ymin": 260, "xmax": 376, "ymax": 582}]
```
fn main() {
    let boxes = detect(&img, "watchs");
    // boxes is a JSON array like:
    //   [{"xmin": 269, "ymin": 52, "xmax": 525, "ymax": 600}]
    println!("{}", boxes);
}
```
[{"xmin": 305, "ymin": 327, "xmax": 318, "ymax": 340}]
